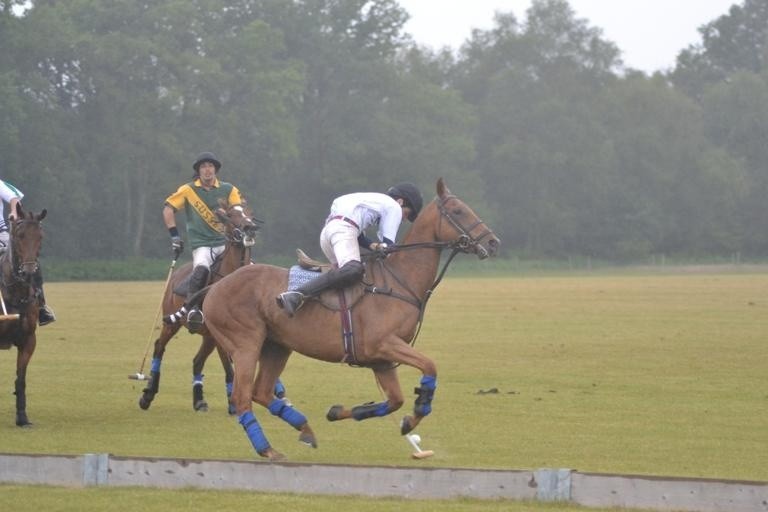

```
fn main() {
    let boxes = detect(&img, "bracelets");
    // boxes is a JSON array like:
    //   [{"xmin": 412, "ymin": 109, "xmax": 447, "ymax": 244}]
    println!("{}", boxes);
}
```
[{"xmin": 168, "ymin": 226, "xmax": 178, "ymax": 237}]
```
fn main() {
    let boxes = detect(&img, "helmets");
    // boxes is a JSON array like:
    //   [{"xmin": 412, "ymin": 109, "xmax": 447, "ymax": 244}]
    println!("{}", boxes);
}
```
[
  {"xmin": 193, "ymin": 151, "xmax": 221, "ymax": 172},
  {"xmin": 391, "ymin": 183, "xmax": 423, "ymax": 222}
]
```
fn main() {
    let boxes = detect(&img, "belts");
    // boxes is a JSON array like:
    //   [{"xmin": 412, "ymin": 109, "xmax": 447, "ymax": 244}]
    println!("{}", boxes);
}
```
[{"xmin": 327, "ymin": 215, "xmax": 360, "ymax": 230}]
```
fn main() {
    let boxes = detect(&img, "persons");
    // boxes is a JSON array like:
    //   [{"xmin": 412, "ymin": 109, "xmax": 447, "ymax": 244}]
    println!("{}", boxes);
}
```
[
  {"xmin": 160, "ymin": 151, "xmax": 254, "ymax": 333},
  {"xmin": 274, "ymin": 182, "xmax": 422, "ymax": 318},
  {"xmin": 0, "ymin": 180, "xmax": 56, "ymax": 327}
]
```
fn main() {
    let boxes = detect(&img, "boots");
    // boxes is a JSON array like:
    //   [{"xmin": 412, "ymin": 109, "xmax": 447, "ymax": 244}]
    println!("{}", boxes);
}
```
[
  {"xmin": 188, "ymin": 280, "xmax": 203, "ymax": 321},
  {"xmin": 276, "ymin": 268, "xmax": 337, "ymax": 318},
  {"xmin": 33, "ymin": 270, "xmax": 54, "ymax": 323}
]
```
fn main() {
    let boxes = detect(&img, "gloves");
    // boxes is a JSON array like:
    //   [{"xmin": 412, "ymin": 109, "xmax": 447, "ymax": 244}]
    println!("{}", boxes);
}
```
[{"xmin": 171, "ymin": 239, "xmax": 185, "ymax": 254}]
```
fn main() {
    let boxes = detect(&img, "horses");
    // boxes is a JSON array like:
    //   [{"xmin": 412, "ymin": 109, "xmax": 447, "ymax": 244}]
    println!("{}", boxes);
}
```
[
  {"xmin": 162, "ymin": 178, "xmax": 500, "ymax": 464},
  {"xmin": 139, "ymin": 198, "xmax": 259, "ymax": 411},
  {"xmin": 0, "ymin": 202, "xmax": 48, "ymax": 427}
]
